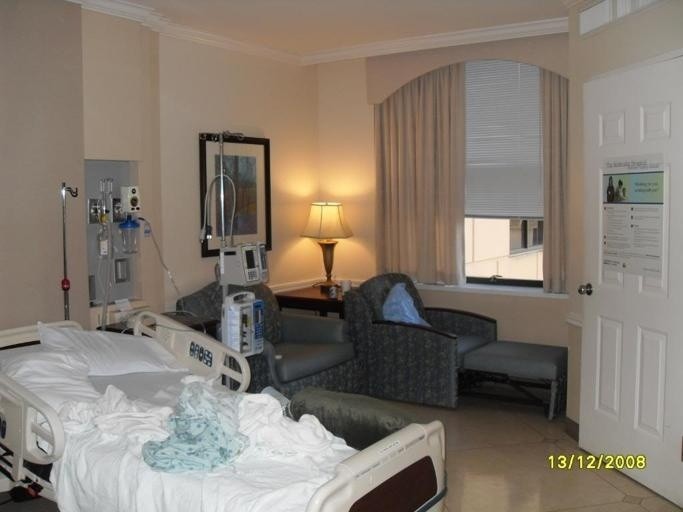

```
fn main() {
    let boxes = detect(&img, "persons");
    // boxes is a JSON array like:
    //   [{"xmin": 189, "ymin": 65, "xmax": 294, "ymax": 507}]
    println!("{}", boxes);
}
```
[
  {"xmin": 604, "ymin": 176, "xmax": 616, "ymax": 204},
  {"xmin": 614, "ymin": 179, "xmax": 629, "ymax": 201}
]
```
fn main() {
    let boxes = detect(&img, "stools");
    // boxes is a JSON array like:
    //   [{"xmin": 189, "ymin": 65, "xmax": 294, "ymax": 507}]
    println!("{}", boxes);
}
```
[{"xmin": 462, "ymin": 337, "xmax": 567, "ymax": 422}]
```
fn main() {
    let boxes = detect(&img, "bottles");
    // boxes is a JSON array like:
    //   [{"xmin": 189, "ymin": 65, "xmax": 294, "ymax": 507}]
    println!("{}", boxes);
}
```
[{"xmin": 328, "ymin": 286, "xmax": 338, "ymax": 298}]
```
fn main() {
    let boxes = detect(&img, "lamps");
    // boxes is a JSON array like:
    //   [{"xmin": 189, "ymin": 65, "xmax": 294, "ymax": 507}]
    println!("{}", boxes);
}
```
[{"xmin": 298, "ymin": 200, "xmax": 354, "ymax": 292}]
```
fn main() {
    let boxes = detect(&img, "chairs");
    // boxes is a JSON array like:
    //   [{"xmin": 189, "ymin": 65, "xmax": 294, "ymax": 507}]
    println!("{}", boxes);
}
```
[
  {"xmin": 346, "ymin": 272, "xmax": 497, "ymax": 409},
  {"xmin": 175, "ymin": 278, "xmax": 355, "ymax": 397}
]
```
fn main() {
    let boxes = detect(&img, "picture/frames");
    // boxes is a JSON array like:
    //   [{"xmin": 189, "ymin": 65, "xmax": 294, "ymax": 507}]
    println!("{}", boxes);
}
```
[{"xmin": 197, "ymin": 131, "xmax": 271, "ymax": 257}]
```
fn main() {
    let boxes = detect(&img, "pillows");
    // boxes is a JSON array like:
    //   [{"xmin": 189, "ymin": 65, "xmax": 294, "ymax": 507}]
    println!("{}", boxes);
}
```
[
  {"xmin": 382, "ymin": 282, "xmax": 431, "ymax": 327},
  {"xmin": 34, "ymin": 316, "xmax": 189, "ymax": 376},
  {"xmin": 3, "ymin": 351, "xmax": 100, "ymax": 426}
]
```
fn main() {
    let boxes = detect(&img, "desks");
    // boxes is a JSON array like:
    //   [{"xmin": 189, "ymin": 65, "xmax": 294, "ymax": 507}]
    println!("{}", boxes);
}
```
[
  {"xmin": 95, "ymin": 311, "xmax": 221, "ymax": 343},
  {"xmin": 275, "ymin": 285, "xmax": 348, "ymax": 323}
]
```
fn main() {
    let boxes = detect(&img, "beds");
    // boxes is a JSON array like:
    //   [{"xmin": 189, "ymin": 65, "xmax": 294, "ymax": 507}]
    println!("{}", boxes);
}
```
[{"xmin": 0, "ymin": 305, "xmax": 448, "ymax": 512}]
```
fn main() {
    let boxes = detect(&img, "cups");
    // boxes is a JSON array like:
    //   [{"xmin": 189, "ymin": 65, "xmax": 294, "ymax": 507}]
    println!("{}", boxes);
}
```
[
  {"xmin": 96, "ymin": 221, "xmax": 139, "ymax": 259},
  {"xmin": 341, "ymin": 280, "xmax": 351, "ymax": 296}
]
